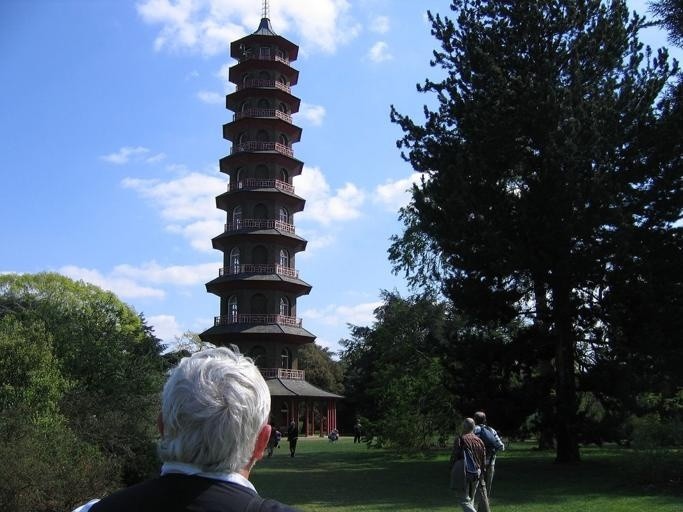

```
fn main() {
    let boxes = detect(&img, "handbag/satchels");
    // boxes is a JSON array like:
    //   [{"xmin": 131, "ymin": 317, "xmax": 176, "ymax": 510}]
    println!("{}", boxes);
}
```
[{"xmin": 450, "ymin": 458, "xmax": 467, "ymax": 491}]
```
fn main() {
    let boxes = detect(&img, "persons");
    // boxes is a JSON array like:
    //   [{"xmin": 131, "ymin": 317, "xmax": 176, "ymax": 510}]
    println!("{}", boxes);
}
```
[
  {"xmin": 472, "ymin": 412, "xmax": 505, "ymax": 512},
  {"xmin": 287, "ymin": 421, "xmax": 298, "ymax": 458},
  {"xmin": 71, "ymin": 346, "xmax": 309, "ymax": 512},
  {"xmin": 447, "ymin": 418, "xmax": 486, "ymax": 512},
  {"xmin": 353, "ymin": 418, "xmax": 361, "ymax": 443},
  {"xmin": 264, "ymin": 414, "xmax": 275, "ymax": 457}
]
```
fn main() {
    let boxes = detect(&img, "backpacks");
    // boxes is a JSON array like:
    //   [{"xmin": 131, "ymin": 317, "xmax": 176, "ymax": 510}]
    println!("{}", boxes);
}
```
[{"xmin": 478, "ymin": 424, "xmax": 498, "ymax": 449}]
